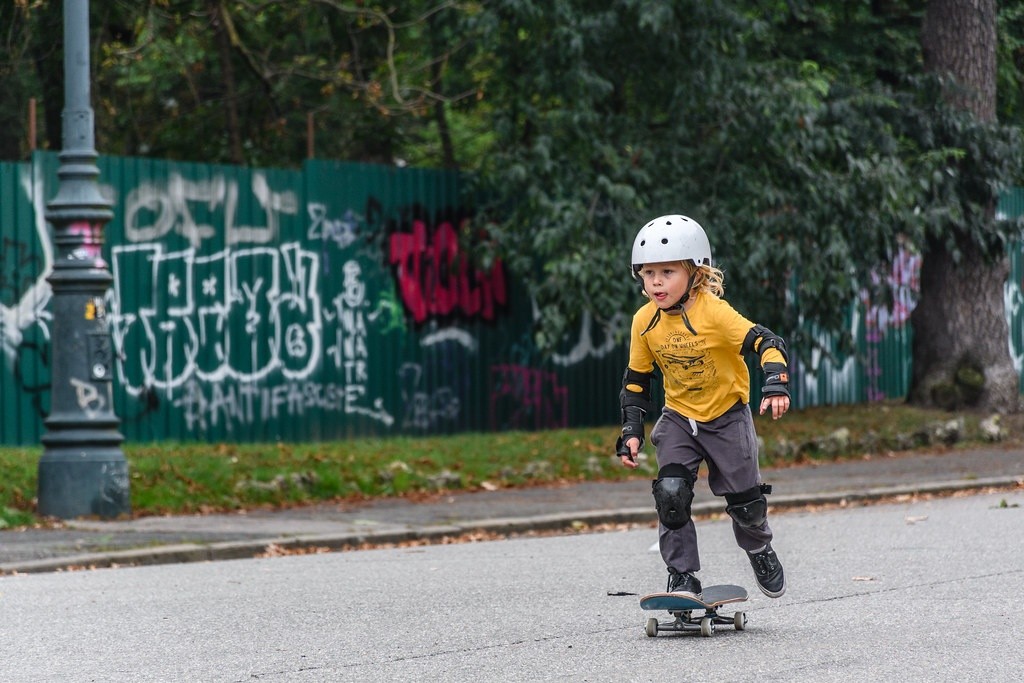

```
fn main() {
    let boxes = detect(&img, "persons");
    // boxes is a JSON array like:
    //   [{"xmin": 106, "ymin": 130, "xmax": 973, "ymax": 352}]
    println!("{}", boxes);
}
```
[{"xmin": 613, "ymin": 212, "xmax": 795, "ymax": 601}]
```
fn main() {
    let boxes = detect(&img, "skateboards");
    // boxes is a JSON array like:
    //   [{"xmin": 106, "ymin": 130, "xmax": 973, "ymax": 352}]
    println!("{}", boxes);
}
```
[{"xmin": 639, "ymin": 583, "xmax": 749, "ymax": 639}]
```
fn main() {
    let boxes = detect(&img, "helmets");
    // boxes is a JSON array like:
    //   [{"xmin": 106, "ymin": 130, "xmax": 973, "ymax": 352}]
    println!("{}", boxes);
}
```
[{"xmin": 630, "ymin": 213, "xmax": 711, "ymax": 278}]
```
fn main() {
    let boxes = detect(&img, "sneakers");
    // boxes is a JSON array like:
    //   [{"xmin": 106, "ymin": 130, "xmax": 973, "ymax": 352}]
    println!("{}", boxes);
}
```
[
  {"xmin": 746, "ymin": 543, "xmax": 786, "ymax": 598},
  {"xmin": 670, "ymin": 571, "xmax": 703, "ymax": 600}
]
